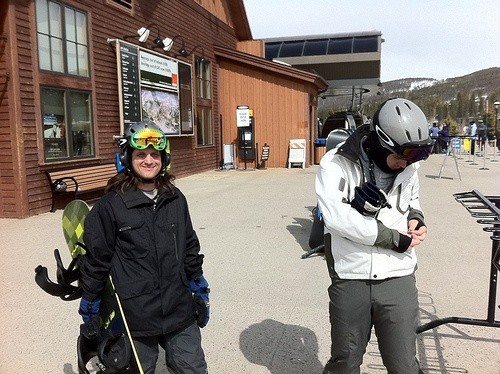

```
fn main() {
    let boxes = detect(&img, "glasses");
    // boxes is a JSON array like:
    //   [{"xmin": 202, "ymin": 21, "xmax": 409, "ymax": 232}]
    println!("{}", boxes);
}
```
[
  {"xmin": 129, "ymin": 128, "xmax": 167, "ymax": 151},
  {"xmin": 397, "ymin": 137, "xmax": 434, "ymax": 163}
]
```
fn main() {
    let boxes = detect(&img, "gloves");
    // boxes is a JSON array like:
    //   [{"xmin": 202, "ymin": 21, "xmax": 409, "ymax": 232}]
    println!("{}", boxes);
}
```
[
  {"xmin": 191, "ymin": 275, "xmax": 209, "ymax": 328},
  {"xmin": 79, "ymin": 298, "xmax": 107, "ymax": 336},
  {"xmin": 352, "ymin": 182, "xmax": 392, "ymax": 216}
]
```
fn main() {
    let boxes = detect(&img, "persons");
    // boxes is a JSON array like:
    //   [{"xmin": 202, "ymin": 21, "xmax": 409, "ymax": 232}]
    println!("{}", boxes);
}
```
[
  {"xmin": 313, "ymin": 97, "xmax": 428, "ymax": 374},
  {"xmin": 467, "ymin": 121, "xmax": 478, "ymax": 155},
  {"xmin": 494, "ymin": 119, "xmax": 500, "ymax": 150},
  {"xmin": 74, "ymin": 130, "xmax": 87, "ymax": 156},
  {"xmin": 77, "ymin": 121, "xmax": 212, "ymax": 374},
  {"xmin": 439, "ymin": 124, "xmax": 449, "ymax": 154},
  {"xmin": 476, "ymin": 119, "xmax": 487, "ymax": 157},
  {"xmin": 428, "ymin": 122, "xmax": 440, "ymax": 155}
]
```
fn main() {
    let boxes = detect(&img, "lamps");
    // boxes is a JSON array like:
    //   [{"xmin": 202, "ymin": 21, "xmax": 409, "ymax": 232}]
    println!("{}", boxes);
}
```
[
  {"xmin": 124, "ymin": 23, "xmax": 164, "ymax": 48},
  {"xmin": 175, "ymin": 44, "xmax": 207, "ymax": 64},
  {"xmin": 151, "ymin": 34, "xmax": 188, "ymax": 57}
]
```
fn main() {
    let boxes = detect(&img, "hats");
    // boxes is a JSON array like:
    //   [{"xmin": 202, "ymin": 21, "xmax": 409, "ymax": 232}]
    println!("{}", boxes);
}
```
[
  {"xmin": 469, "ymin": 121, "xmax": 475, "ymax": 125},
  {"xmin": 478, "ymin": 119, "xmax": 483, "ymax": 123}
]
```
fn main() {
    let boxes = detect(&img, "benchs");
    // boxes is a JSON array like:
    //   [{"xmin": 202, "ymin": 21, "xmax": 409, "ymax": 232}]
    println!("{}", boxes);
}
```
[{"xmin": 46, "ymin": 162, "xmax": 118, "ymax": 212}]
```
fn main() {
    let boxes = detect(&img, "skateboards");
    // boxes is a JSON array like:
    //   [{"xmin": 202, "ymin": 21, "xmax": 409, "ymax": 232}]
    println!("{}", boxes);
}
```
[
  {"xmin": 308, "ymin": 127, "xmax": 352, "ymax": 254},
  {"xmin": 35, "ymin": 200, "xmax": 144, "ymax": 374}
]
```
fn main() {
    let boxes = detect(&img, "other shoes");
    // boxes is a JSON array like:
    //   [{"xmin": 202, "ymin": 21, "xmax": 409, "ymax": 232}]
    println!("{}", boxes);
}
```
[{"xmin": 442, "ymin": 149, "xmax": 446, "ymax": 153}]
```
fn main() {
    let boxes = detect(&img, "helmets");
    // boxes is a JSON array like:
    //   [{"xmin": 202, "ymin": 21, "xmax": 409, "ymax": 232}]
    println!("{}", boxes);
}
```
[
  {"xmin": 77, "ymin": 328, "xmax": 131, "ymax": 374},
  {"xmin": 369, "ymin": 97, "xmax": 429, "ymax": 155},
  {"xmin": 124, "ymin": 120, "xmax": 171, "ymax": 167}
]
